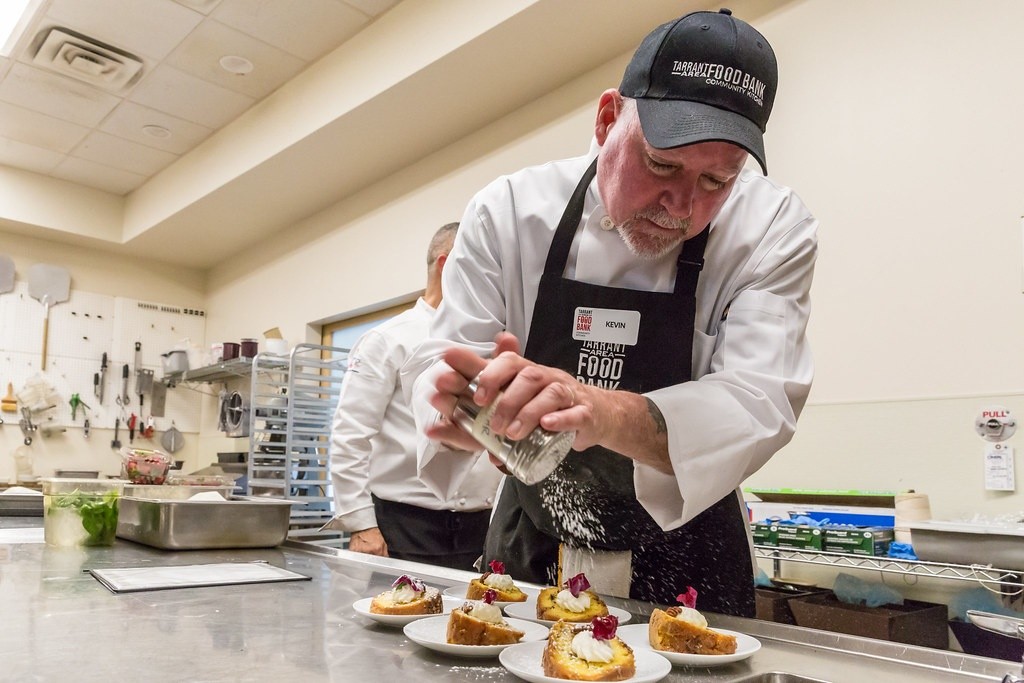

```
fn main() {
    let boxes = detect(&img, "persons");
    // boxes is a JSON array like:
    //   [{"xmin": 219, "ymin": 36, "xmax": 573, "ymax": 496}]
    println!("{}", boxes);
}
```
[
  {"xmin": 403, "ymin": 9, "xmax": 819, "ymax": 618},
  {"xmin": 327, "ymin": 222, "xmax": 506, "ymax": 571}
]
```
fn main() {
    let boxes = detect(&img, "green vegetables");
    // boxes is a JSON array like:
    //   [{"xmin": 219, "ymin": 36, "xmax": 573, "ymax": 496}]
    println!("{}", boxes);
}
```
[{"xmin": 47, "ymin": 492, "xmax": 118, "ymax": 546}]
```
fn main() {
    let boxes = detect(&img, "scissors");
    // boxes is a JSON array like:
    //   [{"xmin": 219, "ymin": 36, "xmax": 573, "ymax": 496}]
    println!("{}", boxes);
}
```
[{"xmin": 115, "ymin": 394, "xmax": 130, "ymax": 419}]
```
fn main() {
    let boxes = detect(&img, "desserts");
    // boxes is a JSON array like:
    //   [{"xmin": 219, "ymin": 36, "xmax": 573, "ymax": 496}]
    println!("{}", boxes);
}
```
[
  {"xmin": 542, "ymin": 615, "xmax": 635, "ymax": 681},
  {"xmin": 466, "ymin": 559, "xmax": 528, "ymax": 602},
  {"xmin": 537, "ymin": 573, "xmax": 608, "ymax": 623},
  {"xmin": 650, "ymin": 588, "xmax": 737, "ymax": 655},
  {"xmin": 447, "ymin": 589, "xmax": 524, "ymax": 646},
  {"xmin": 369, "ymin": 574, "xmax": 443, "ymax": 615}
]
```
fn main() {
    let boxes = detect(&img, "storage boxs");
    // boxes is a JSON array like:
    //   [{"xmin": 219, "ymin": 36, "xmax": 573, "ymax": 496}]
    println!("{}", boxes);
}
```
[
  {"xmin": 35, "ymin": 476, "xmax": 133, "ymax": 548},
  {"xmin": 160, "ymin": 350, "xmax": 190, "ymax": 374}
]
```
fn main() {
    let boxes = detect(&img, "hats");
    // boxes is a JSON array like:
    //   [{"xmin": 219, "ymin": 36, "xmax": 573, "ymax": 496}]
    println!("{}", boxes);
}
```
[{"xmin": 619, "ymin": 9, "xmax": 778, "ymax": 176}]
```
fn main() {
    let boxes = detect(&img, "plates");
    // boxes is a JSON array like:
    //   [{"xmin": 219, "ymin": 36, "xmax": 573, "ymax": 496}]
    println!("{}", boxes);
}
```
[
  {"xmin": 616, "ymin": 624, "xmax": 762, "ymax": 667},
  {"xmin": 353, "ymin": 596, "xmax": 463, "ymax": 628},
  {"xmin": 504, "ymin": 602, "xmax": 631, "ymax": 629},
  {"xmin": 499, "ymin": 640, "xmax": 672, "ymax": 683},
  {"xmin": 403, "ymin": 616, "xmax": 550, "ymax": 658},
  {"xmin": 444, "ymin": 586, "xmax": 540, "ymax": 611}
]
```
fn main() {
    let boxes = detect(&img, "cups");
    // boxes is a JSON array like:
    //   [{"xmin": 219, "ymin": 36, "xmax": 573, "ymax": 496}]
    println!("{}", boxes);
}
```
[
  {"xmin": 241, "ymin": 338, "xmax": 259, "ymax": 358},
  {"xmin": 223, "ymin": 342, "xmax": 240, "ymax": 361}
]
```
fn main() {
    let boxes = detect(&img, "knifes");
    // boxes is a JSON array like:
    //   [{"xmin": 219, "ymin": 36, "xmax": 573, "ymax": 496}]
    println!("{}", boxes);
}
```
[
  {"xmin": 94, "ymin": 373, "xmax": 99, "ymax": 395},
  {"xmin": 100, "ymin": 352, "xmax": 108, "ymax": 404},
  {"xmin": 122, "ymin": 364, "xmax": 129, "ymax": 409},
  {"xmin": 134, "ymin": 342, "xmax": 142, "ymax": 376},
  {"xmin": 139, "ymin": 394, "xmax": 143, "ymax": 418}
]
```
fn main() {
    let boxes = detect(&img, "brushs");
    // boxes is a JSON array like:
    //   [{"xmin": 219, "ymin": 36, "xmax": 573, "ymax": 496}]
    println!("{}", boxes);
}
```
[{"xmin": 1, "ymin": 382, "xmax": 18, "ymax": 411}]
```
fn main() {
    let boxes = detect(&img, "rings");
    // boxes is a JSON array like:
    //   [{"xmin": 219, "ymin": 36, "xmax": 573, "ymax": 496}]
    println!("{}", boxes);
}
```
[{"xmin": 564, "ymin": 385, "xmax": 574, "ymax": 407}]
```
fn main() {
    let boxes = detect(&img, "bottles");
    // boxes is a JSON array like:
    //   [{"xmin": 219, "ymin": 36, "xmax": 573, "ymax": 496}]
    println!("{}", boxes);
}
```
[{"xmin": 451, "ymin": 371, "xmax": 576, "ymax": 486}]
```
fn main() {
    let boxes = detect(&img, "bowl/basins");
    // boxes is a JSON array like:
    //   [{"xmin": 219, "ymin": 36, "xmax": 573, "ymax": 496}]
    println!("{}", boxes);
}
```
[{"xmin": 38, "ymin": 477, "xmax": 132, "ymax": 547}]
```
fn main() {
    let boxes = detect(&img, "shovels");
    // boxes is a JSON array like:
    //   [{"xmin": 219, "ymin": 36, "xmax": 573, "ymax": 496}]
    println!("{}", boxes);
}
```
[{"xmin": 111, "ymin": 419, "xmax": 121, "ymax": 450}]
